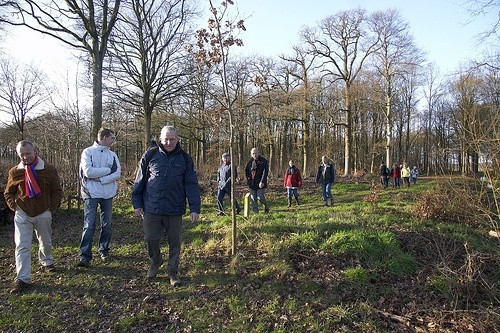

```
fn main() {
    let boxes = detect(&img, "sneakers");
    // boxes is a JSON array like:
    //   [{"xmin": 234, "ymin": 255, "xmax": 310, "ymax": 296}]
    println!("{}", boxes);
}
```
[
  {"xmin": 147, "ymin": 260, "xmax": 164, "ymax": 278},
  {"xmin": 168, "ymin": 271, "xmax": 182, "ymax": 286}
]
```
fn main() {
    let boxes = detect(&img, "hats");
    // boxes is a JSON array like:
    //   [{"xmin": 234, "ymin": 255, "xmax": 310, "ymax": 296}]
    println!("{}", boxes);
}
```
[{"xmin": 221, "ymin": 153, "xmax": 230, "ymax": 157}]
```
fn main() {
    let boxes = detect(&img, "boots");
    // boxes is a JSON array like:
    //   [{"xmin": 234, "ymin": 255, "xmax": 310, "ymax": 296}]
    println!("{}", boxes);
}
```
[
  {"xmin": 286, "ymin": 197, "xmax": 292, "ymax": 207},
  {"xmin": 294, "ymin": 197, "xmax": 299, "ymax": 207}
]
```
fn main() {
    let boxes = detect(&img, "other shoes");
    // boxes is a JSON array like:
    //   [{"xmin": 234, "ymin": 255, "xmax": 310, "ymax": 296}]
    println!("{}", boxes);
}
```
[
  {"xmin": 217, "ymin": 211, "xmax": 225, "ymax": 216},
  {"xmin": 265, "ymin": 207, "xmax": 270, "ymax": 213},
  {"xmin": 45, "ymin": 263, "xmax": 55, "ymax": 273},
  {"xmin": 323, "ymin": 202, "xmax": 329, "ymax": 205},
  {"xmin": 393, "ymin": 186, "xmax": 400, "ymax": 188},
  {"xmin": 78, "ymin": 257, "xmax": 89, "ymax": 265},
  {"xmin": 100, "ymin": 252, "xmax": 110, "ymax": 262},
  {"xmin": 331, "ymin": 197, "xmax": 334, "ymax": 204},
  {"xmin": 10, "ymin": 278, "xmax": 28, "ymax": 293},
  {"xmin": 235, "ymin": 208, "xmax": 241, "ymax": 214}
]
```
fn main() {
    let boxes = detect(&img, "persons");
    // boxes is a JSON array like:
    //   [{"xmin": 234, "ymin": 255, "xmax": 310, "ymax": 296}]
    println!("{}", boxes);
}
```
[
  {"xmin": 74, "ymin": 127, "xmax": 121, "ymax": 267},
  {"xmin": 131, "ymin": 126, "xmax": 201, "ymax": 287},
  {"xmin": 245, "ymin": 147, "xmax": 270, "ymax": 214},
  {"xmin": 315, "ymin": 156, "xmax": 336, "ymax": 206},
  {"xmin": 3, "ymin": 140, "xmax": 63, "ymax": 293},
  {"xmin": 217, "ymin": 153, "xmax": 241, "ymax": 216},
  {"xmin": 284, "ymin": 160, "xmax": 302, "ymax": 208},
  {"xmin": 379, "ymin": 160, "xmax": 419, "ymax": 189}
]
